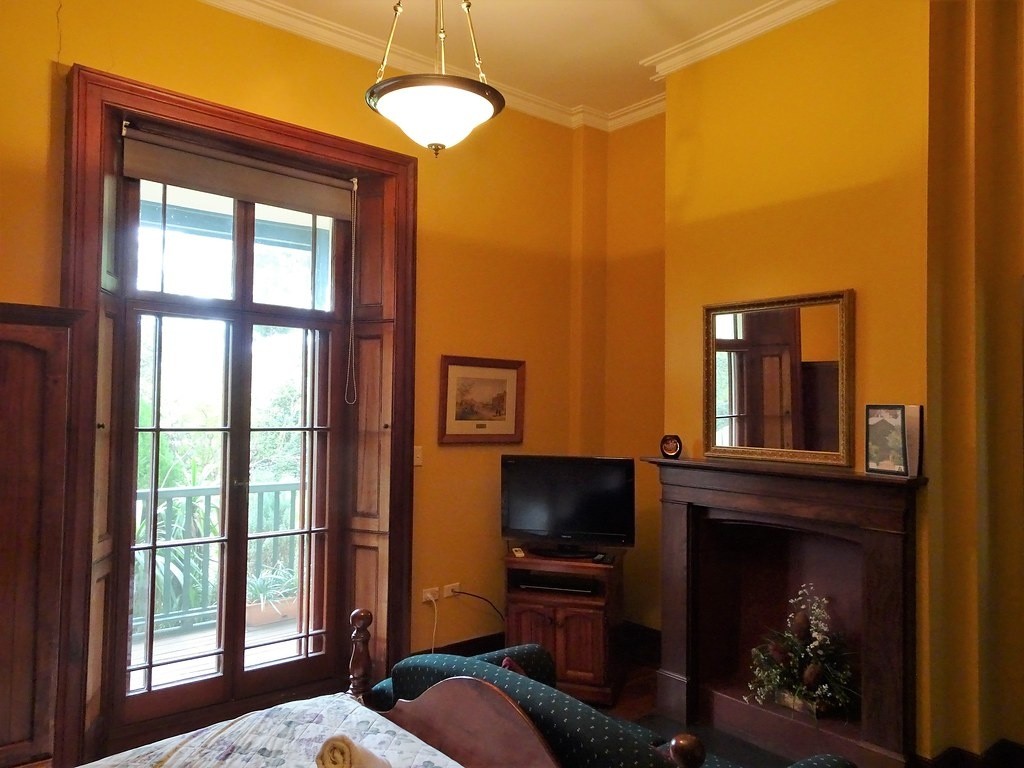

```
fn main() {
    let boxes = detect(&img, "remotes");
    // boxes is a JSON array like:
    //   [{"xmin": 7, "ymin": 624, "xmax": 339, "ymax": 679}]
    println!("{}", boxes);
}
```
[
  {"xmin": 512, "ymin": 548, "xmax": 525, "ymax": 558},
  {"xmin": 592, "ymin": 553, "xmax": 617, "ymax": 564}
]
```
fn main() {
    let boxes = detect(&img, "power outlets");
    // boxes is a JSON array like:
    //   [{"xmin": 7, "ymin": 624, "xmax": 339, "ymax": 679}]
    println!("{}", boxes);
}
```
[
  {"xmin": 422, "ymin": 586, "xmax": 439, "ymax": 602},
  {"xmin": 443, "ymin": 582, "xmax": 460, "ymax": 598}
]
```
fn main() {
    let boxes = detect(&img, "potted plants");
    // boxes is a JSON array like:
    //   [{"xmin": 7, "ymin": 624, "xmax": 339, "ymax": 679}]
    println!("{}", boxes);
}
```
[{"xmin": 245, "ymin": 560, "xmax": 299, "ymax": 627}]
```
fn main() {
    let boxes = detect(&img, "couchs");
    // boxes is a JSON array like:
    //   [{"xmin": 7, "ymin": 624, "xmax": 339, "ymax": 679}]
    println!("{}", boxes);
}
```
[{"xmin": 350, "ymin": 607, "xmax": 860, "ymax": 768}]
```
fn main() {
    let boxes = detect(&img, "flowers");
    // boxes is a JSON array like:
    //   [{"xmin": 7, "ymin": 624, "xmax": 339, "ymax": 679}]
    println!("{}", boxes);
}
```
[{"xmin": 741, "ymin": 582, "xmax": 867, "ymax": 727}]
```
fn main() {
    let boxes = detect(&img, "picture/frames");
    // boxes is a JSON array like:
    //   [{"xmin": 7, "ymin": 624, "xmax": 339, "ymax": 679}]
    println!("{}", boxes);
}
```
[{"xmin": 438, "ymin": 354, "xmax": 527, "ymax": 445}]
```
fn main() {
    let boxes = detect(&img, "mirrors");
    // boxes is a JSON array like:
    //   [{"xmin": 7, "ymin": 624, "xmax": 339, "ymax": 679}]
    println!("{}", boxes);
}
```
[{"xmin": 702, "ymin": 288, "xmax": 857, "ymax": 466}]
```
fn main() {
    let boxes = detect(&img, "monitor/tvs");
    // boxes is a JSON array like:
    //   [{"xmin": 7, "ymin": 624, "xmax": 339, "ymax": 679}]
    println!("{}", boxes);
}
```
[{"xmin": 501, "ymin": 454, "xmax": 635, "ymax": 557}]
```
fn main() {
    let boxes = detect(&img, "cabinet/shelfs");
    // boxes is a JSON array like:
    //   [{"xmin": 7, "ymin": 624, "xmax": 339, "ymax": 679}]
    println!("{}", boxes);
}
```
[{"xmin": 500, "ymin": 543, "xmax": 627, "ymax": 710}]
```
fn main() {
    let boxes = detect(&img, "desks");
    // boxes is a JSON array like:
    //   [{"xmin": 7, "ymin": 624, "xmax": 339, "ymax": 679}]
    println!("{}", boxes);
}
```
[{"xmin": 75, "ymin": 690, "xmax": 467, "ymax": 768}]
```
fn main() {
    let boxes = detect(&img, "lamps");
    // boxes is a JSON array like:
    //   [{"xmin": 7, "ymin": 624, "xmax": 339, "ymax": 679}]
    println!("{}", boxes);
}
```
[{"xmin": 364, "ymin": 0, "xmax": 506, "ymax": 159}]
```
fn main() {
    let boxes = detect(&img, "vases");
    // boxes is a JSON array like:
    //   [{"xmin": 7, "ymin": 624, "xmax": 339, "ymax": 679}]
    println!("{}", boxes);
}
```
[{"xmin": 781, "ymin": 690, "xmax": 830, "ymax": 717}]
